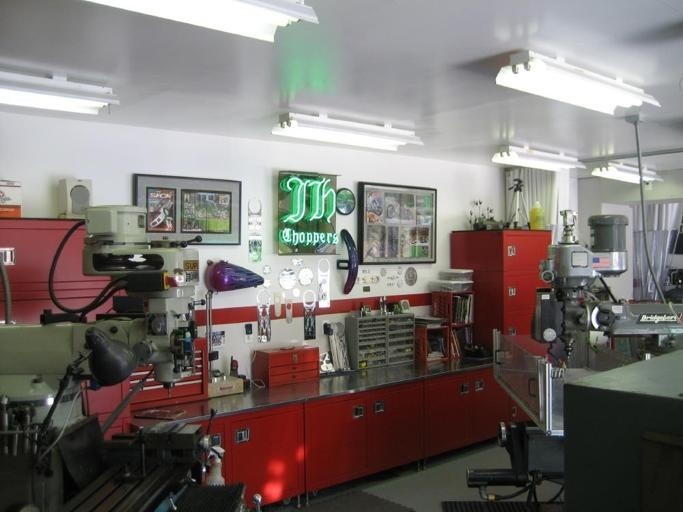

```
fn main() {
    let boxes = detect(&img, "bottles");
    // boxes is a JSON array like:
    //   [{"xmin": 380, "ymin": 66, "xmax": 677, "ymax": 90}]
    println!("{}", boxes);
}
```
[{"xmin": 529, "ymin": 200, "xmax": 544, "ymax": 230}]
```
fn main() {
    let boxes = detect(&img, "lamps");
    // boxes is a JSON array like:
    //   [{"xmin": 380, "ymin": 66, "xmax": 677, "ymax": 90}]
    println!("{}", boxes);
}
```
[
  {"xmin": 2, "ymin": 71, "xmax": 120, "ymax": 114},
  {"xmin": 272, "ymin": 112, "xmax": 424, "ymax": 151},
  {"xmin": 496, "ymin": 49, "xmax": 661, "ymax": 115},
  {"xmin": 492, "ymin": 145, "xmax": 586, "ymax": 171},
  {"xmin": 38, "ymin": 325, "xmax": 139, "ymax": 456},
  {"xmin": 592, "ymin": 162, "xmax": 657, "ymax": 184},
  {"xmin": 94, "ymin": 0, "xmax": 320, "ymax": 42}
]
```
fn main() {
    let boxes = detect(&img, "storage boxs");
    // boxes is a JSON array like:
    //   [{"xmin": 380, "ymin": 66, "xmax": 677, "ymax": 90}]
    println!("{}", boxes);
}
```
[
  {"xmin": 429, "ymin": 268, "xmax": 474, "ymax": 292},
  {"xmin": 253, "ymin": 345, "xmax": 320, "ymax": 377},
  {"xmin": 416, "ymin": 291, "xmax": 477, "ymax": 363}
]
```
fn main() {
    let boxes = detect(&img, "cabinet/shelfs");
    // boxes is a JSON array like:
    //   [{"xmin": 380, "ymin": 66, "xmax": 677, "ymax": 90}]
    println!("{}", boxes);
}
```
[
  {"xmin": 450, "ymin": 228, "xmax": 554, "ymax": 435},
  {"xmin": 193, "ymin": 365, "xmax": 510, "ymax": 512},
  {"xmin": 0, "ymin": 219, "xmax": 131, "ymax": 444}
]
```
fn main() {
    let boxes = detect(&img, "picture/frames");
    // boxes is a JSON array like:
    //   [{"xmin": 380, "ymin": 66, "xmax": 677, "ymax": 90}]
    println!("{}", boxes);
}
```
[
  {"xmin": 181, "ymin": 189, "xmax": 232, "ymax": 233},
  {"xmin": 357, "ymin": 181, "xmax": 437, "ymax": 263},
  {"xmin": 145, "ymin": 186, "xmax": 176, "ymax": 233},
  {"xmin": 131, "ymin": 173, "xmax": 241, "ymax": 244},
  {"xmin": 336, "ymin": 189, "xmax": 355, "ymax": 215}
]
